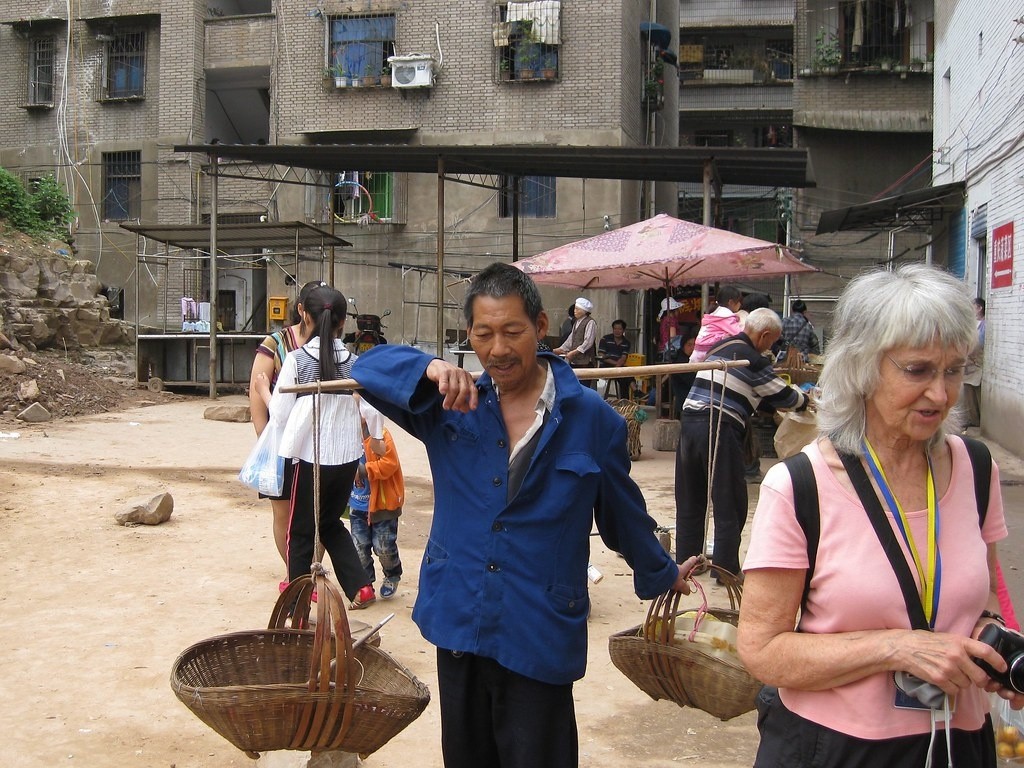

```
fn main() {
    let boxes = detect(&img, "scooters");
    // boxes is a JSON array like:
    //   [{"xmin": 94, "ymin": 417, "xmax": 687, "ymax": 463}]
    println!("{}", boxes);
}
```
[{"xmin": 346, "ymin": 297, "xmax": 391, "ymax": 356}]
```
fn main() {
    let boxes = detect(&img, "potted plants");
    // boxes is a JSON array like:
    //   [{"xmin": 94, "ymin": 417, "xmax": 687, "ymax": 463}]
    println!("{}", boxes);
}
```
[
  {"xmin": 541, "ymin": 58, "xmax": 556, "ymax": 79},
  {"xmin": 378, "ymin": 67, "xmax": 391, "ymax": 85},
  {"xmin": 516, "ymin": 20, "xmax": 540, "ymax": 79},
  {"xmin": 499, "ymin": 59, "xmax": 511, "ymax": 82},
  {"xmin": 797, "ymin": 23, "xmax": 933, "ymax": 74},
  {"xmin": 322, "ymin": 66, "xmax": 335, "ymax": 89},
  {"xmin": 364, "ymin": 64, "xmax": 375, "ymax": 85},
  {"xmin": 350, "ymin": 72, "xmax": 360, "ymax": 88},
  {"xmin": 644, "ymin": 78, "xmax": 655, "ymax": 109}
]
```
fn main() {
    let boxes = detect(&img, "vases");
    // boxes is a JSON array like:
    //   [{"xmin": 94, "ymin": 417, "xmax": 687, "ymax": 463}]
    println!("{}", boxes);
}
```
[{"xmin": 336, "ymin": 76, "xmax": 347, "ymax": 89}]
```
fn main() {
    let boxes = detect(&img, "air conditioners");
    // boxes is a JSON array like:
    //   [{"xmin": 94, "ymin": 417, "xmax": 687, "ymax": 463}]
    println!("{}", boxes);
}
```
[{"xmin": 391, "ymin": 60, "xmax": 434, "ymax": 89}]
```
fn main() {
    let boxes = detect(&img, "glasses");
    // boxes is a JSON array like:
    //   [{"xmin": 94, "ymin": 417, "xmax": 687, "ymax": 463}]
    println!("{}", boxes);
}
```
[{"xmin": 880, "ymin": 352, "xmax": 978, "ymax": 382}]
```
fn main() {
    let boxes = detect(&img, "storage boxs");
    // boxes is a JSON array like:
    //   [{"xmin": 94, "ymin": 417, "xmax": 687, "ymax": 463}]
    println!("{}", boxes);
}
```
[{"xmin": 357, "ymin": 315, "xmax": 381, "ymax": 332}]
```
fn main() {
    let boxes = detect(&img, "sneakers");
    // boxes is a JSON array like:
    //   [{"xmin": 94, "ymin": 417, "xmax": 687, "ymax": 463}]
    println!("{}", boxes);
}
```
[
  {"xmin": 370, "ymin": 583, "xmax": 377, "ymax": 602},
  {"xmin": 633, "ymin": 389, "xmax": 650, "ymax": 400},
  {"xmin": 379, "ymin": 575, "xmax": 401, "ymax": 599}
]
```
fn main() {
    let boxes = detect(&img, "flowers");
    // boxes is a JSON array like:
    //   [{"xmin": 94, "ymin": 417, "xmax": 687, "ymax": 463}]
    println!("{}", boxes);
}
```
[
  {"xmin": 330, "ymin": 44, "xmax": 347, "ymax": 76},
  {"xmin": 653, "ymin": 49, "xmax": 667, "ymax": 91}
]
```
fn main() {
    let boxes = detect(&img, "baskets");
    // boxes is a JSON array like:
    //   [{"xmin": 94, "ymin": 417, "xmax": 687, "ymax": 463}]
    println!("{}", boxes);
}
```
[
  {"xmin": 609, "ymin": 562, "xmax": 766, "ymax": 722},
  {"xmin": 170, "ymin": 574, "xmax": 431, "ymax": 761}
]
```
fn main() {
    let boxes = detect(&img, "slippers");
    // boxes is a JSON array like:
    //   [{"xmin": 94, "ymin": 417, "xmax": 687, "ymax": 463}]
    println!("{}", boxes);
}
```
[{"xmin": 960, "ymin": 422, "xmax": 978, "ymax": 427}]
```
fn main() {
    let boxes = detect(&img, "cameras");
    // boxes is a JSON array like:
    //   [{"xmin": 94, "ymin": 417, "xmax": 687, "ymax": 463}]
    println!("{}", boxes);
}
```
[{"xmin": 967, "ymin": 609, "xmax": 1024, "ymax": 694}]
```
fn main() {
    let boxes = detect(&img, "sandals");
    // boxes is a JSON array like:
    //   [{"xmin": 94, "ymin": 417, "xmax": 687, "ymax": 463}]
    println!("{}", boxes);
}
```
[
  {"xmin": 348, "ymin": 583, "xmax": 376, "ymax": 610},
  {"xmin": 285, "ymin": 615, "xmax": 310, "ymax": 630}
]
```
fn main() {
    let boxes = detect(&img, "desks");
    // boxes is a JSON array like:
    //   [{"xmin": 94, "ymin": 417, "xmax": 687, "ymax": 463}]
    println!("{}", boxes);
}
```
[
  {"xmin": 450, "ymin": 350, "xmax": 603, "ymax": 370},
  {"xmin": 138, "ymin": 333, "xmax": 271, "ymax": 384}
]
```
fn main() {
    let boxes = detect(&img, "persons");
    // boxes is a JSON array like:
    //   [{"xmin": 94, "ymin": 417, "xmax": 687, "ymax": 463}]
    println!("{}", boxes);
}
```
[
  {"xmin": 963, "ymin": 298, "xmax": 985, "ymax": 428},
  {"xmin": 657, "ymin": 297, "xmax": 685, "ymax": 419},
  {"xmin": 537, "ymin": 297, "xmax": 598, "ymax": 393},
  {"xmin": 736, "ymin": 265, "xmax": 1024, "ymax": 768},
  {"xmin": 675, "ymin": 307, "xmax": 816, "ymax": 584},
  {"xmin": 598, "ymin": 319, "xmax": 649, "ymax": 402},
  {"xmin": 353, "ymin": 415, "xmax": 405, "ymax": 600},
  {"xmin": 247, "ymin": 280, "xmax": 327, "ymax": 603},
  {"xmin": 673, "ymin": 287, "xmax": 820, "ymax": 424},
  {"xmin": 255, "ymin": 285, "xmax": 383, "ymax": 610},
  {"xmin": 348, "ymin": 263, "xmax": 695, "ymax": 768}
]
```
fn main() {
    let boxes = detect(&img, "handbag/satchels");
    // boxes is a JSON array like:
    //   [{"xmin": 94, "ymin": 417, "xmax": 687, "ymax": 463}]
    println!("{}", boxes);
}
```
[
  {"xmin": 995, "ymin": 697, "xmax": 1024, "ymax": 768},
  {"xmin": 236, "ymin": 415, "xmax": 286, "ymax": 497},
  {"xmin": 772, "ymin": 337, "xmax": 789, "ymax": 354}
]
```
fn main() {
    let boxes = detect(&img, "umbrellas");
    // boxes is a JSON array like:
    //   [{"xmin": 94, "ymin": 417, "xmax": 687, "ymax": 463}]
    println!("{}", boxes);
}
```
[{"xmin": 465, "ymin": 214, "xmax": 819, "ymax": 422}]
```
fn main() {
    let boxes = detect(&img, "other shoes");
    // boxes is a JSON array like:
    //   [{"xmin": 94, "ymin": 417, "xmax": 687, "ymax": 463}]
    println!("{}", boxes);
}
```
[
  {"xmin": 716, "ymin": 567, "xmax": 746, "ymax": 586},
  {"xmin": 279, "ymin": 577, "xmax": 289, "ymax": 593},
  {"xmin": 311, "ymin": 592, "xmax": 317, "ymax": 603},
  {"xmin": 691, "ymin": 558, "xmax": 710, "ymax": 575}
]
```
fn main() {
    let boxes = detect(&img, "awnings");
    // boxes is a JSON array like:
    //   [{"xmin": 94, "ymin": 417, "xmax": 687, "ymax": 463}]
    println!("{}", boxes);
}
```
[
  {"xmin": 75, "ymin": 12, "xmax": 161, "ymax": 21},
  {"xmin": 640, "ymin": 21, "xmax": 677, "ymax": 64},
  {"xmin": 0, "ymin": 16, "xmax": 68, "ymax": 26},
  {"xmin": 815, "ymin": 182, "xmax": 963, "ymax": 234},
  {"xmin": 295, "ymin": 126, "xmax": 419, "ymax": 140}
]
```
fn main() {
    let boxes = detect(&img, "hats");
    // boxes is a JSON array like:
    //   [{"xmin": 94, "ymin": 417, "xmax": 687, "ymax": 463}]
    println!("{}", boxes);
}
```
[
  {"xmin": 575, "ymin": 297, "xmax": 593, "ymax": 314},
  {"xmin": 658, "ymin": 297, "xmax": 685, "ymax": 319},
  {"xmin": 970, "ymin": 298, "xmax": 985, "ymax": 308}
]
